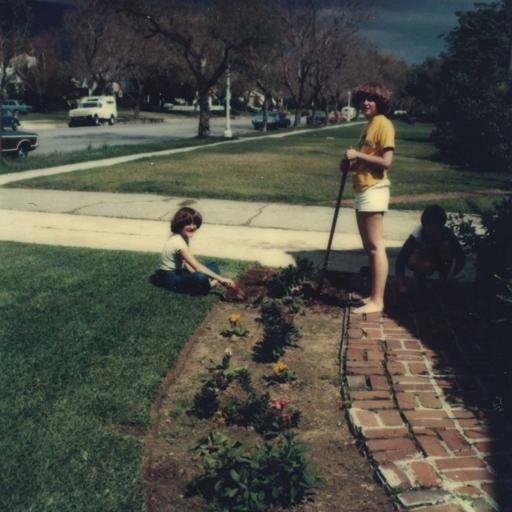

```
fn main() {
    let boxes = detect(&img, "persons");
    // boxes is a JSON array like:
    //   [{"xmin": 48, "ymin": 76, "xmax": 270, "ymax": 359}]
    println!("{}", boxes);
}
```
[
  {"xmin": 339, "ymin": 80, "xmax": 396, "ymax": 314},
  {"xmin": 151, "ymin": 207, "xmax": 237, "ymax": 294},
  {"xmin": 394, "ymin": 205, "xmax": 466, "ymax": 296}
]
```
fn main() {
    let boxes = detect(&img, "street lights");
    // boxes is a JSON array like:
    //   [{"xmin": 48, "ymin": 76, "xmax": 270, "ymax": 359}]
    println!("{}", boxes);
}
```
[{"xmin": 347, "ymin": 90, "xmax": 351, "ymax": 123}]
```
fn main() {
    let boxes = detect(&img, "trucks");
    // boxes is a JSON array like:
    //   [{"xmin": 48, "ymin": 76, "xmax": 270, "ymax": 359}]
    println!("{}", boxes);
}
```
[{"xmin": 67, "ymin": 94, "xmax": 119, "ymax": 127}]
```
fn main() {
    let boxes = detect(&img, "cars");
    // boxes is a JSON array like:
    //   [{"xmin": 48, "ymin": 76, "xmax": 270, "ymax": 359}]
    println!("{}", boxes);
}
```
[
  {"xmin": 0, "ymin": 97, "xmax": 40, "ymax": 161},
  {"xmin": 250, "ymin": 108, "xmax": 408, "ymax": 132}
]
